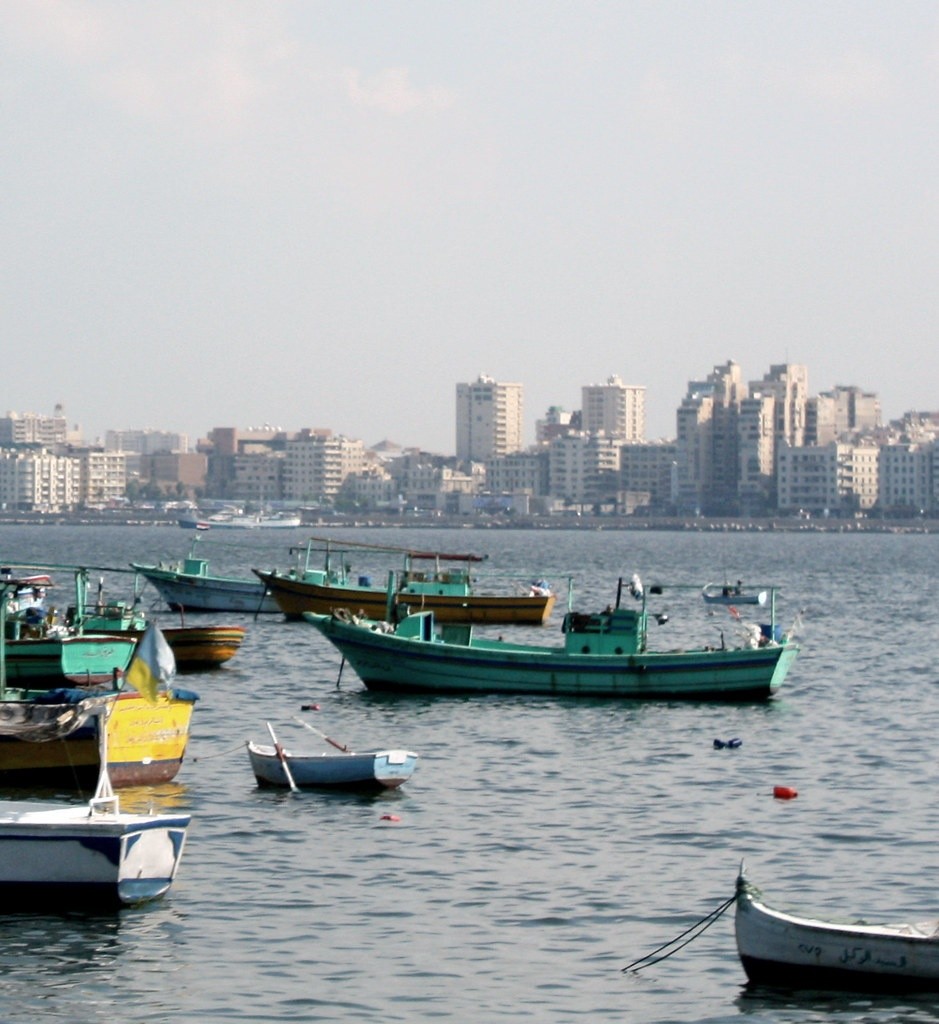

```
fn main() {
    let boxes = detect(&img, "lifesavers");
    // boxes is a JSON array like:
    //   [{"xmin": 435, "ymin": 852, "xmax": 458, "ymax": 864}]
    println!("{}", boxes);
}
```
[{"xmin": 333, "ymin": 607, "xmax": 348, "ymax": 621}]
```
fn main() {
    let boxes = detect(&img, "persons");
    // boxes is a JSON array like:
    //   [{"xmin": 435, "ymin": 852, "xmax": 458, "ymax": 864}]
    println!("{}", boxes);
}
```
[
  {"xmin": 723, "ymin": 578, "xmax": 742, "ymax": 597},
  {"xmin": 758, "ymin": 632, "xmax": 778, "ymax": 647}
]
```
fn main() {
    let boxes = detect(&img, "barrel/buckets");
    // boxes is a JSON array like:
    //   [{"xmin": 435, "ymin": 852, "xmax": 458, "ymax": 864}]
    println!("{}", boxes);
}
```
[
  {"xmin": 761, "ymin": 623, "xmax": 782, "ymax": 641},
  {"xmin": 358, "ymin": 576, "xmax": 372, "ymax": 587}
]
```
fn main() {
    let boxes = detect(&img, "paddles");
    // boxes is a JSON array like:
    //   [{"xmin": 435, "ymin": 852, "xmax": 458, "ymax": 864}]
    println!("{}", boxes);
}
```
[
  {"xmin": 295, "ymin": 717, "xmax": 354, "ymax": 755},
  {"xmin": 2, "ymin": 697, "xmax": 59, "ymax": 706},
  {"xmin": 267, "ymin": 722, "xmax": 303, "ymax": 799}
]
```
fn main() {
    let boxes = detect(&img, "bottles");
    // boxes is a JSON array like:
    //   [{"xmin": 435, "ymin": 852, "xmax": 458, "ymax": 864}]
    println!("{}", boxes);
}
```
[{"xmin": 773, "ymin": 785, "xmax": 798, "ymax": 799}]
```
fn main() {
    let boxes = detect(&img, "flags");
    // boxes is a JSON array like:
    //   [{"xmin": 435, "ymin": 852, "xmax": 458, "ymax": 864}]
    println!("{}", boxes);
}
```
[{"xmin": 125, "ymin": 622, "xmax": 175, "ymax": 708}]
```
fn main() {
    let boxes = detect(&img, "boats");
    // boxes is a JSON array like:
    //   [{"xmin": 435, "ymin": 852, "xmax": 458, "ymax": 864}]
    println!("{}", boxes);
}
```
[
  {"xmin": 251, "ymin": 546, "xmax": 556, "ymax": 627},
  {"xmin": 0, "ymin": 564, "xmax": 138, "ymax": 686},
  {"xmin": 247, "ymin": 741, "xmax": 419, "ymax": 791},
  {"xmin": 0, "ymin": 681, "xmax": 197, "ymax": 786},
  {"xmin": 0, "ymin": 708, "xmax": 191, "ymax": 906},
  {"xmin": 176, "ymin": 496, "xmax": 302, "ymax": 529},
  {"xmin": 155, "ymin": 626, "xmax": 246, "ymax": 668},
  {"xmin": 131, "ymin": 536, "xmax": 419, "ymax": 614},
  {"xmin": 302, "ymin": 575, "xmax": 806, "ymax": 697},
  {"xmin": 734, "ymin": 858, "xmax": 939, "ymax": 994}
]
